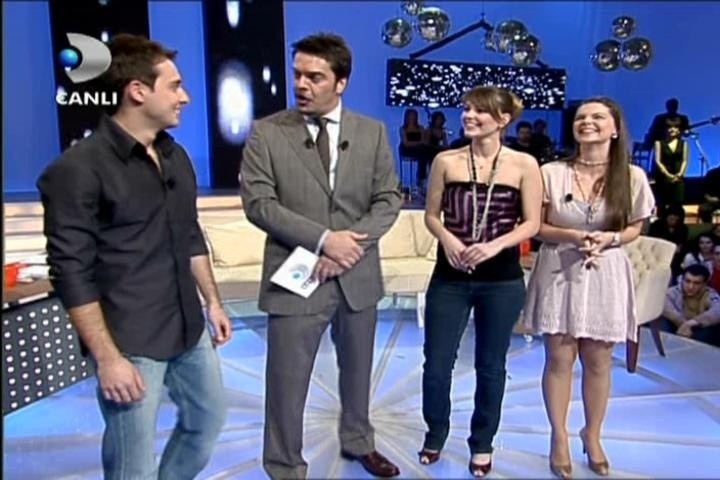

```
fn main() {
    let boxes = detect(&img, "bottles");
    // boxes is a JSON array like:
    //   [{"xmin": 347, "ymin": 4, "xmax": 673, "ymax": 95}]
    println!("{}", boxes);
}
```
[{"xmin": 4, "ymin": 265, "xmax": 18, "ymax": 286}]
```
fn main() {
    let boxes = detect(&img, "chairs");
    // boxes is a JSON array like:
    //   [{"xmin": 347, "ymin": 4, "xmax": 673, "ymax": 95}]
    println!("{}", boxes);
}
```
[
  {"xmin": 621, "ymin": 235, "xmax": 678, "ymax": 373},
  {"xmin": 398, "ymin": 143, "xmax": 419, "ymax": 203}
]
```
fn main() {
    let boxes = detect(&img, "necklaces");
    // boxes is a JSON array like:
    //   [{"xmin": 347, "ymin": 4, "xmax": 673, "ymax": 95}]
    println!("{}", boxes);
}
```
[
  {"xmin": 466, "ymin": 141, "xmax": 505, "ymax": 272},
  {"xmin": 571, "ymin": 158, "xmax": 607, "ymax": 225}
]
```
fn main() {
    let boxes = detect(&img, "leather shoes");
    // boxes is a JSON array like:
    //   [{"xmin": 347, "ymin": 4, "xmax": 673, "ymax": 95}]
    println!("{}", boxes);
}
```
[
  {"xmin": 418, "ymin": 448, "xmax": 440, "ymax": 464},
  {"xmin": 340, "ymin": 448, "xmax": 399, "ymax": 477},
  {"xmin": 469, "ymin": 461, "xmax": 491, "ymax": 477}
]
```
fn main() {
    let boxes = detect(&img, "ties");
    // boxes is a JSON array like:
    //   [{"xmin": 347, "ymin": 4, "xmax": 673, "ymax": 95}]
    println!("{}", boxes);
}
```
[{"xmin": 313, "ymin": 117, "xmax": 329, "ymax": 176}]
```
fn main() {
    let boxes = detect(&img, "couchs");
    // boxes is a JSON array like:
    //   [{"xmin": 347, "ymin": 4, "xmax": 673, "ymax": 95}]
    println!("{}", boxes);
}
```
[{"xmin": 193, "ymin": 209, "xmax": 444, "ymax": 329}]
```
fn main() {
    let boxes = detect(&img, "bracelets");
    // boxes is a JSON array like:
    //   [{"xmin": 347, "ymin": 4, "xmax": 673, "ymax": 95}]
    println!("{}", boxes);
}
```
[{"xmin": 611, "ymin": 232, "xmax": 621, "ymax": 246}]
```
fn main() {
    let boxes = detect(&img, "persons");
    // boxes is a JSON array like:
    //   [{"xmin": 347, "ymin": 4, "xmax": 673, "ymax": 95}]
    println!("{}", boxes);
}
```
[
  {"xmin": 424, "ymin": 111, "xmax": 450, "ymax": 151},
  {"xmin": 35, "ymin": 34, "xmax": 231, "ymax": 480},
  {"xmin": 399, "ymin": 109, "xmax": 436, "ymax": 186},
  {"xmin": 539, "ymin": 97, "xmax": 656, "ymax": 480},
  {"xmin": 507, "ymin": 121, "xmax": 539, "ymax": 166},
  {"xmin": 529, "ymin": 119, "xmax": 551, "ymax": 153},
  {"xmin": 644, "ymin": 98, "xmax": 688, "ymax": 150},
  {"xmin": 654, "ymin": 118, "xmax": 688, "ymax": 217},
  {"xmin": 416, "ymin": 85, "xmax": 544, "ymax": 478},
  {"xmin": 239, "ymin": 32, "xmax": 401, "ymax": 480},
  {"xmin": 649, "ymin": 205, "xmax": 720, "ymax": 347}
]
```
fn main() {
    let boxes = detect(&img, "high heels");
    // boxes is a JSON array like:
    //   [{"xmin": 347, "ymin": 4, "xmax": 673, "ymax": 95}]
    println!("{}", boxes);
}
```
[
  {"xmin": 578, "ymin": 428, "xmax": 609, "ymax": 476},
  {"xmin": 550, "ymin": 429, "xmax": 572, "ymax": 480}
]
